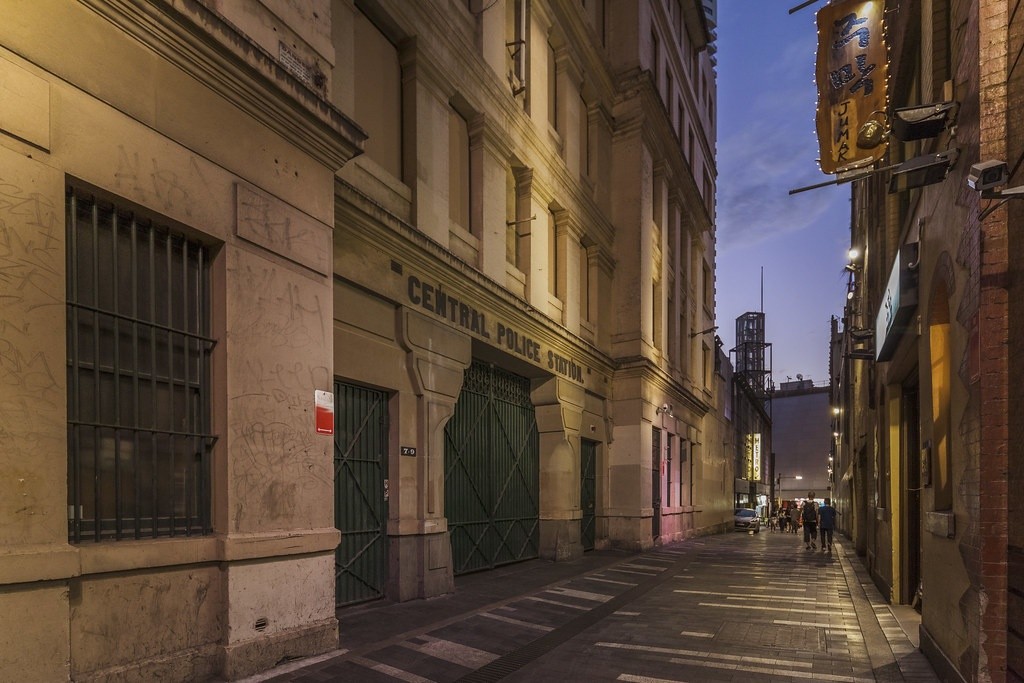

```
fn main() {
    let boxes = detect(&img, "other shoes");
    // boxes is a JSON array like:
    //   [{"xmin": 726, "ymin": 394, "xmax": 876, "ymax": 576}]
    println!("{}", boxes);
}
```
[
  {"xmin": 811, "ymin": 542, "xmax": 817, "ymax": 549},
  {"xmin": 823, "ymin": 545, "xmax": 826, "ymax": 550},
  {"xmin": 828, "ymin": 543, "xmax": 831, "ymax": 551},
  {"xmin": 806, "ymin": 545, "xmax": 811, "ymax": 550}
]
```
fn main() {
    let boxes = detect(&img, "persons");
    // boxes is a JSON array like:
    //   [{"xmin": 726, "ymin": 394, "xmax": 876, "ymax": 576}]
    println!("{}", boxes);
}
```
[
  {"xmin": 801, "ymin": 491, "xmax": 819, "ymax": 552},
  {"xmin": 818, "ymin": 498, "xmax": 836, "ymax": 552},
  {"xmin": 769, "ymin": 503, "xmax": 803, "ymax": 534}
]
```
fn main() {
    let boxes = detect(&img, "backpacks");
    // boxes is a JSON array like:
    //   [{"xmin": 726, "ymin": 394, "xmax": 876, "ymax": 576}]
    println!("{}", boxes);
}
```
[{"xmin": 803, "ymin": 501, "xmax": 816, "ymax": 520}]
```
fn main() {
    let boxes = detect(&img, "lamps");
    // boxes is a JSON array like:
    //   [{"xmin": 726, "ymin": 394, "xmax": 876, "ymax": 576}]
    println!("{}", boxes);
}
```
[
  {"xmin": 846, "ymin": 348, "xmax": 875, "ymax": 360},
  {"xmin": 848, "ymin": 327, "xmax": 874, "ymax": 339},
  {"xmin": 657, "ymin": 404, "xmax": 673, "ymax": 418},
  {"xmin": 891, "ymin": 100, "xmax": 958, "ymax": 142},
  {"xmin": 856, "ymin": 110, "xmax": 893, "ymax": 149},
  {"xmin": 889, "ymin": 148, "xmax": 961, "ymax": 195}
]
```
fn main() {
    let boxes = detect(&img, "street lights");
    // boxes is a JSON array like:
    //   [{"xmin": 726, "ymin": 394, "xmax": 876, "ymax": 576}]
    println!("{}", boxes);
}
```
[{"xmin": 779, "ymin": 473, "xmax": 802, "ymax": 509}]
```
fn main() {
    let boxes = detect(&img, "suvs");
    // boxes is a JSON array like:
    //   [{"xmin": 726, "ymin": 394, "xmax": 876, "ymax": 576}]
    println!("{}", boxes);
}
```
[{"xmin": 734, "ymin": 508, "xmax": 760, "ymax": 534}]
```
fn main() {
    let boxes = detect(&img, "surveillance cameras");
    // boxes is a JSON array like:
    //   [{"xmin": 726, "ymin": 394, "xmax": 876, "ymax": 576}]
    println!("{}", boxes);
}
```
[
  {"xmin": 662, "ymin": 404, "xmax": 668, "ymax": 410},
  {"xmin": 966, "ymin": 160, "xmax": 1008, "ymax": 191}
]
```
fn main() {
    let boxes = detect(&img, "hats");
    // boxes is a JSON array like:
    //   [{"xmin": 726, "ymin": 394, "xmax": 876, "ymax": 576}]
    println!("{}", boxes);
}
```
[{"xmin": 824, "ymin": 498, "xmax": 830, "ymax": 504}]
